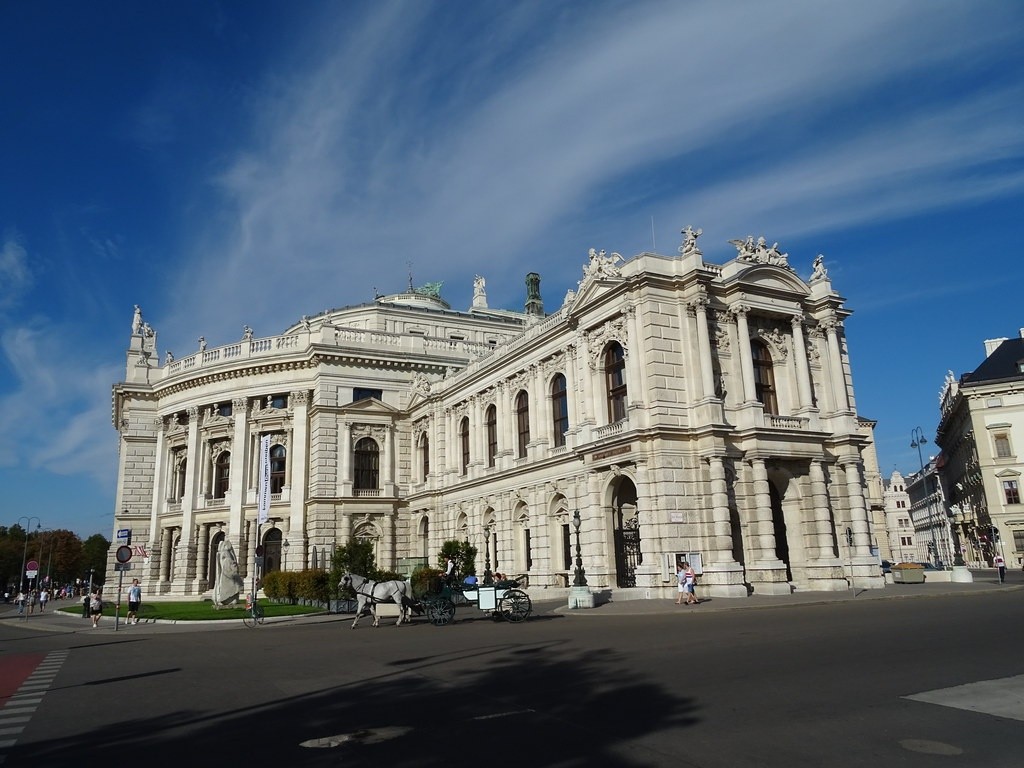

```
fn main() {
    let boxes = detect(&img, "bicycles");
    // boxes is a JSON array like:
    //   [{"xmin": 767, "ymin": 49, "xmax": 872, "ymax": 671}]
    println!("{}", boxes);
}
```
[{"xmin": 242, "ymin": 599, "xmax": 265, "ymax": 628}]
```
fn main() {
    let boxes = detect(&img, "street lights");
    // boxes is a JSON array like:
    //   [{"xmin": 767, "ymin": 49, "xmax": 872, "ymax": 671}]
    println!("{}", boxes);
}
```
[
  {"xmin": 483, "ymin": 526, "xmax": 494, "ymax": 587},
  {"xmin": 17, "ymin": 517, "xmax": 41, "ymax": 592},
  {"xmin": 572, "ymin": 508, "xmax": 588, "ymax": 586},
  {"xmin": 43, "ymin": 527, "xmax": 60, "ymax": 576},
  {"xmin": 283, "ymin": 538, "xmax": 290, "ymax": 571},
  {"xmin": 911, "ymin": 426, "xmax": 942, "ymax": 571}
]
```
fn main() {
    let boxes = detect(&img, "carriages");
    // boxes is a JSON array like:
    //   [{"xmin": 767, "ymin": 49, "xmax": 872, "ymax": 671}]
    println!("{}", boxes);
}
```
[{"xmin": 337, "ymin": 568, "xmax": 533, "ymax": 630}]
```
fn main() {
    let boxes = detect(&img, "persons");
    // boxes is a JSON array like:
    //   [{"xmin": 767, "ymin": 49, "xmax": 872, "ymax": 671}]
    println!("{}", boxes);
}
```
[
  {"xmin": 771, "ymin": 329, "xmax": 783, "ymax": 349},
  {"xmin": 125, "ymin": 579, "xmax": 141, "ymax": 624},
  {"xmin": 301, "ymin": 315, "xmax": 310, "ymax": 330},
  {"xmin": 681, "ymin": 225, "xmax": 700, "ymax": 251},
  {"xmin": 166, "ymin": 350, "xmax": 174, "ymax": 362},
  {"xmin": 171, "ymin": 414, "xmax": 179, "ymax": 431},
  {"xmin": 474, "ymin": 275, "xmax": 486, "ymax": 294},
  {"xmin": 323, "ymin": 310, "xmax": 332, "ymax": 323},
  {"xmin": 412, "ymin": 373, "xmax": 431, "ymax": 392},
  {"xmin": 993, "ymin": 552, "xmax": 1006, "ymax": 582},
  {"xmin": 262, "ymin": 396, "xmax": 274, "ymax": 415},
  {"xmin": 440, "ymin": 556, "xmax": 456, "ymax": 592},
  {"xmin": 737, "ymin": 237, "xmax": 789, "ymax": 267},
  {"xmin": 577, "ymin": 248, "xmax": 622, "ymax": 288},
  {"xmin": 675, "ymin": 562, "xmax": 701, "ymax": 605},
  {"xmin": 197, "ymin": 336, "xmax": 206, "ymax": 351},
  {"xmin": 0, "ymin": 584, "xmax": 103, "ymax": 614},
  {"xmin": 464, "ymin": 573, "xmax": 478, "ymax": 584},
  {"xmin": 809, "ymin": 254, "xmax": 828, "ymax": 282},
  {"xmin": 494, "ymin": 572, "xmax": 507, "ymax": 587},
  {"xmin": 89, "ymin": 594, "xmax": 102, "ymax": 627},
  {"xmin": 242, "ymin": 325, "xmax": 254, "ymax": 339},
  {"xmin": 132, "ymin": 305, "xmax": 154, "ymax": 337},
  {"xmin": 210, "ymin": 404, "xmax": 220, "ymax": 422}
]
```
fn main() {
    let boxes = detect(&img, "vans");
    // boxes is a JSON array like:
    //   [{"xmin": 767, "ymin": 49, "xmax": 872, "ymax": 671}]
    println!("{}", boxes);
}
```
[{"xmin": 882, "ymin": 559, "xmax": 897, "ymax": 573}]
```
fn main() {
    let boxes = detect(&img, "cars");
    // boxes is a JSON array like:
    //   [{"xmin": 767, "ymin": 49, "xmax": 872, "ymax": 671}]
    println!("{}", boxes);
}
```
[{"xmin": 917, "ymin": 562, "xmax": 939, "ymax": 570}]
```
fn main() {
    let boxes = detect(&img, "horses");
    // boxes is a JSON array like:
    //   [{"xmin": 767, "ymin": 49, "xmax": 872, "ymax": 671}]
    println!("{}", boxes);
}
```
[{"xmin": 337, "ymin": 568, "xmax": 413, "ymax": 630}]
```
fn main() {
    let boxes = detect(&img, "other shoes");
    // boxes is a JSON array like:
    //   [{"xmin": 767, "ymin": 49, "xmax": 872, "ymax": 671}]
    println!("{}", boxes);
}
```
[
  {"xmin": 125, "ymin": 618, "xmax": 129, "ymax": 624},
  {"xmin": 93, "ymin": 624, "xmax": 97, "ymax": 628},
  {"xmin": 132, "ymin": 622, "xmax": 136, "ymax": 625}
]
